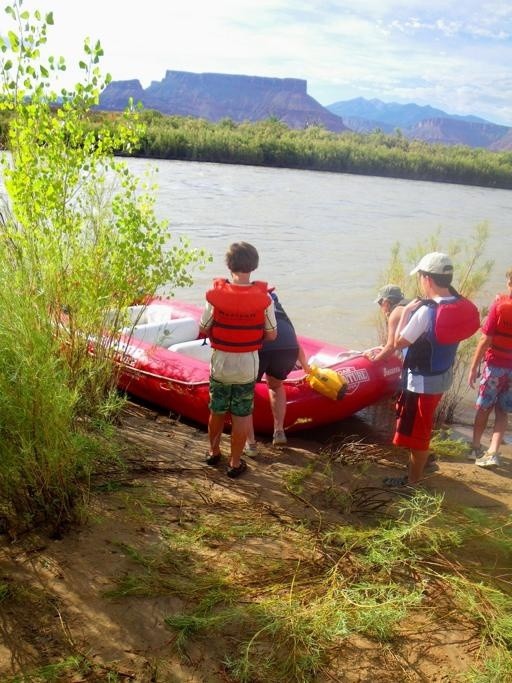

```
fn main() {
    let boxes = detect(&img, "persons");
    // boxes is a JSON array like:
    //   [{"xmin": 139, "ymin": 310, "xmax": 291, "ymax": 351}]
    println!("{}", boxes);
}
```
[
  {"xmin": 244, "ymin": 293, "xmax": 309, "ymax": 457},
  {"xmin": 467, "ymin": 267, "xmax": 512, "ymax": 468},
  {"xmin": 384, "ymin": 252, "xmax": 480, "ymax": 486},
  {"xmin": 366, "ymin": 284, "xmax": 440, "ymax": 472},
  {"xmin": 198, "ymin": 241, "xmax": 278, "ymax": 477}
]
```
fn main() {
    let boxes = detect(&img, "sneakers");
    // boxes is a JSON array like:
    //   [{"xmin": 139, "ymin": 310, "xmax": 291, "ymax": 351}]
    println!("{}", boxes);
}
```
[
  {"xmin": 472, "ymin": 451, "xmax": 500, "ymax": 468},
  {"xmin": 271, "ymin": 429, "xmax": 288, "ymax": 447},
  {"xmin": 205, "ymin": 446, "xmax": 222, "ymax": 465},
  {"xmin": 463, "ymin": 444, "xmax": 486, "ymax": 461},
  {"xmin": 243, "ymin": 442, "xmax": 261, "ymax": 457},
  {"xmin": 226, "ymin": 457, "xmax": 247, "ymax": 478}
]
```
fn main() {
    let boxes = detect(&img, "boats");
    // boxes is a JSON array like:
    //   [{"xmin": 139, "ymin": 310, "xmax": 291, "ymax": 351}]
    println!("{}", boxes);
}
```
[{"xmin": 16, "ymin": 262, "xmax": 409, "ymax": 444}]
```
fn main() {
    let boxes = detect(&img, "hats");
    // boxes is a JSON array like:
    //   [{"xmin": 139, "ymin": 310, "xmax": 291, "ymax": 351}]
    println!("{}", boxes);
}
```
[
  {"xmin": 373, "ymin": 285, "xmax": 402, "ymax": 304},
  {"xmin": 410, "ymin": 252, "xmax": 454, "ymax": 276}
]
